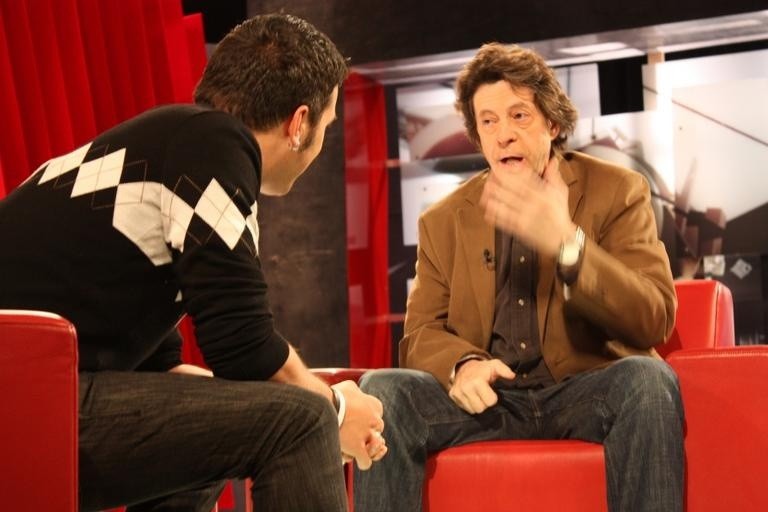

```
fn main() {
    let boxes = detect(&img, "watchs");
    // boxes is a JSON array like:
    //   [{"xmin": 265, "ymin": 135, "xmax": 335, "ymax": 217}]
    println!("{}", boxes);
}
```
[{"xmin": 554, "ymin": 226, "xmax": 585, "ymax": 267}]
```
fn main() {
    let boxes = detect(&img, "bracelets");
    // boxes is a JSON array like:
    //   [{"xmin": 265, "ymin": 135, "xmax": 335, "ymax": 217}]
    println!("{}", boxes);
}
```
[{"xmin": 329, "ymin": 385, "xmax": 346, "ymax": 428}]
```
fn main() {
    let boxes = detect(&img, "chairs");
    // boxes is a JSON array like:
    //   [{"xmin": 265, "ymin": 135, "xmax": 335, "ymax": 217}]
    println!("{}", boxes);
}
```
[
  {"xmin": 0, "ymin": 301, "xmax": 83, "ymax": 510},
  {"xmin": 253, "ymin": 273, "xmax": 768, "ymax": 510}
]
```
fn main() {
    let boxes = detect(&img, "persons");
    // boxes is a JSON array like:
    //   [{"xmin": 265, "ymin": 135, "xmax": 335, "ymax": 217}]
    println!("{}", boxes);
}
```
[
  {"xmin": 352, "ymin": 40, "xmax": 688, "ymax": 512},
  {"xmin": 1, "ymin": 12, "xmax": 389, "ymax": 512}
]
